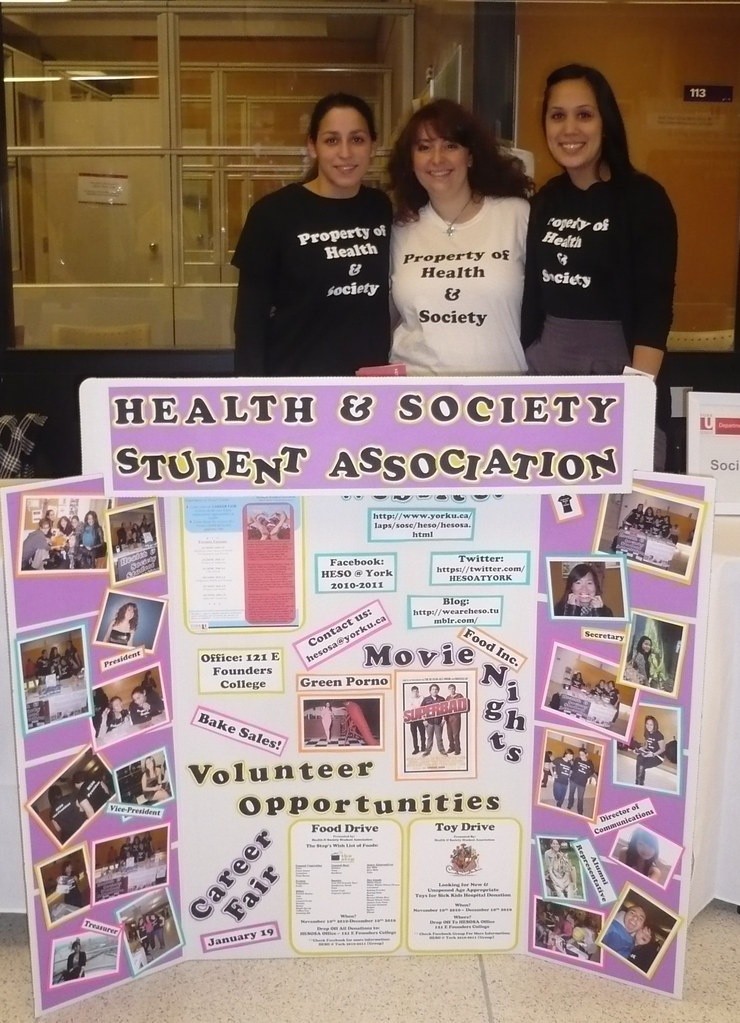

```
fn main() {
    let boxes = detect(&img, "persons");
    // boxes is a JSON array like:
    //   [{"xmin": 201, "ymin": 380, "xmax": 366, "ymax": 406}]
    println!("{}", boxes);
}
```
[
  {"xmin": 22, "ymin": 508, "xmax": 104, "ymax": 570},
  {"xmin": 103, "ymin": 602, "xmax": 138, "ymax": 646},
  {"xmin": 520, "ymin": 63, "xmax": 678, "ymax": 472},
  {"xmin": 602, "ymin": 903, "xmax": 659, "ymax": 974},
  {"xmin": 229, "ymin": 92, "xmax": 392, "ymax": 377},
  {"xmin": 117, "ymin": 515, "xmax": 156, "ymax": 548},
  {"xmin": 25, "ymin": 639, "xmax": 82, "ymax": 685},
  {"xmin": 321, "ymin": 700, "xmax": 333, "ymax": 744},
  {"xmin": 65, "ymin": 911, "xmax": 164, "ymax": 981},
  {"xmin": 619, "ymin": 825, "xmax": 661, "ymax": 883},
  {"xmin": 545, "ymin": 839, "xmax": 572, "ymax": 898},
  {"xmin": 383, "ymin": 98, "xmax": 532, "ymax": 377},
  {"xmin": 550, "ymin": 498, "xmax": 696, "ymax": 816},
  {"xmin": 537, "ymin": 899, "xmax": 598, "ymax": 961},
  {"xmin": 60, "ymin": 861, "xmax": 81, "ymax": 909},
  {"xmin": 410, "ymin": 684, "xmax": 464, "ymax": 756},
  {"xmin": 47, "ymin": 670, "xmax": 171, "ymax": 838},
  {"xmin": 119, "ymin": 832, "xmax": 151, "ymax": 862}
]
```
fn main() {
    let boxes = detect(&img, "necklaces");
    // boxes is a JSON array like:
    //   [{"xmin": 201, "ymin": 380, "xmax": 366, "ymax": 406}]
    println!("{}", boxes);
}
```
[{"xmin": 430, "ymin": 197, "xmax": 473, "ymax": 237}]
